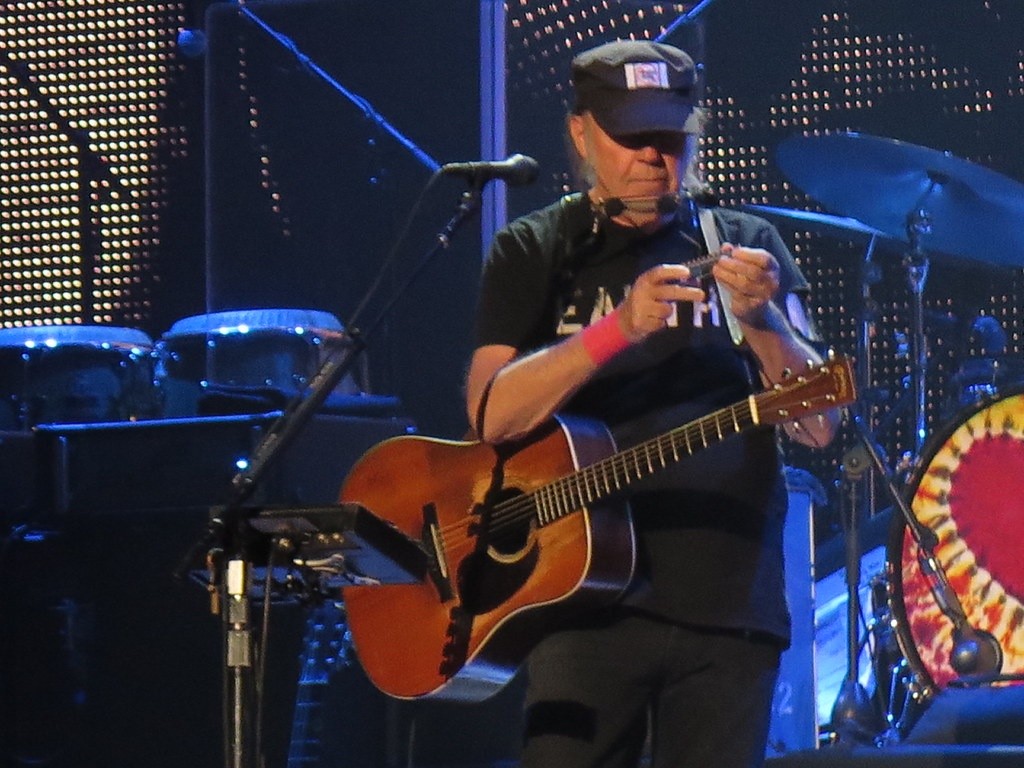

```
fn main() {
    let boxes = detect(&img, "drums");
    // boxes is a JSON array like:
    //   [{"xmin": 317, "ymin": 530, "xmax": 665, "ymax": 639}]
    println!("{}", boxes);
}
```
[{"xmin": 886, "ymin": 386, "xmax": 1024, "ymax": 708}]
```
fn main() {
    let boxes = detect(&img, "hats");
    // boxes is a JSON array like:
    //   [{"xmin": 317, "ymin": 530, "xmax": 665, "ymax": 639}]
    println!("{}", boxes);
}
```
[{"xmin": 571, "ymin": 40, "xmax": 700, "ymax": 136}]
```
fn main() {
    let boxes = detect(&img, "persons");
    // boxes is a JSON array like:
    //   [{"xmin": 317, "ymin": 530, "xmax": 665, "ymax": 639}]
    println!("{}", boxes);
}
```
[{"xmin": 466, "ymin": 40, "xmax": 843, "ymax": 768}]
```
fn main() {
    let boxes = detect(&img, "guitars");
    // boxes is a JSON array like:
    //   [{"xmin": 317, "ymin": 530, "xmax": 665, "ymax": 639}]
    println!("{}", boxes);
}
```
[{"xmin": 331, "ymin": 344, "xmax": 865, "ymax": 704}]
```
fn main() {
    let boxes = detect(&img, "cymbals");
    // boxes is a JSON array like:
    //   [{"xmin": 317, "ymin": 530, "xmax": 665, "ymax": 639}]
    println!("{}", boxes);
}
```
[
  {"xmin": 737, "ymin": 202, "xmax": 896, "ymax": 245},
  {"xmin": 775, "ymin": 130, "xmax": 1024, "ymax": 270}
]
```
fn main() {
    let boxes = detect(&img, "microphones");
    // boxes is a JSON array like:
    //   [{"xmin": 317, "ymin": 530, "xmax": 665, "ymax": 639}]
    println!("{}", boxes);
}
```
[
  {"xmin": 917, "ymin": 543, "xmax": 997, "ymax": 676},
  {"xmin": 442, "ymin": 154, "xmax": 540, "ymax": 186}
]
